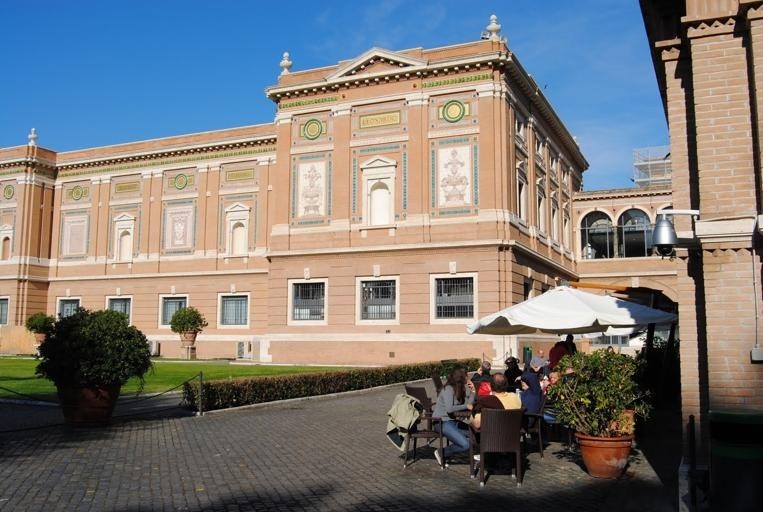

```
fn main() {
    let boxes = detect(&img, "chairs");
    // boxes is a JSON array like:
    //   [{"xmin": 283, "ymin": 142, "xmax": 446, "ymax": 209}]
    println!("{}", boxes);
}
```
[{"xmin": 403, "ymin": 371, "xmax": 546, "ymax": 488}]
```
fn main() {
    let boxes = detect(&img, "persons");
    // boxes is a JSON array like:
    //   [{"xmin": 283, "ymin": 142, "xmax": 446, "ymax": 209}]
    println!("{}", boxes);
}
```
[{"xmin": 429, "ymin": 333, "xmax": 581, "ymax": 476}]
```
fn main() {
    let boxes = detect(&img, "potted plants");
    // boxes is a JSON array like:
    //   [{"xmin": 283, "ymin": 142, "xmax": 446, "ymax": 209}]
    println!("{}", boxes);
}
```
[
  {"xmin": 26, "ymin": 304, "xmax": 209, "ymax": 424},
  {"xmin": 546, "ymin": 345, "xmax": 653, "ymax": 479}
]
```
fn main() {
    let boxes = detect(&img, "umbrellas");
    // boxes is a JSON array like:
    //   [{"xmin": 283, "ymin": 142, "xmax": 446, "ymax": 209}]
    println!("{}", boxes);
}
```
[{"xmin": 467, "ymin": 282, "xmax": 677, "ymax": 347}]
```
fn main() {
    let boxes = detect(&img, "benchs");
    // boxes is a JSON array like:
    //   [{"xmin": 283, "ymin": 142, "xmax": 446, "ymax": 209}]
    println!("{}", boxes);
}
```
[{"xmin": 441, "ymin": 359, "xmax": 467, "ymax": 379}]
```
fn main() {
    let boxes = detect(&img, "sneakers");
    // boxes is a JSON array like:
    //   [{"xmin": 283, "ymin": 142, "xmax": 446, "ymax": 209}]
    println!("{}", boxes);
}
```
[{"xmin": 434, "ymin": 449, "xmax": 443, "ymax": 466}]
